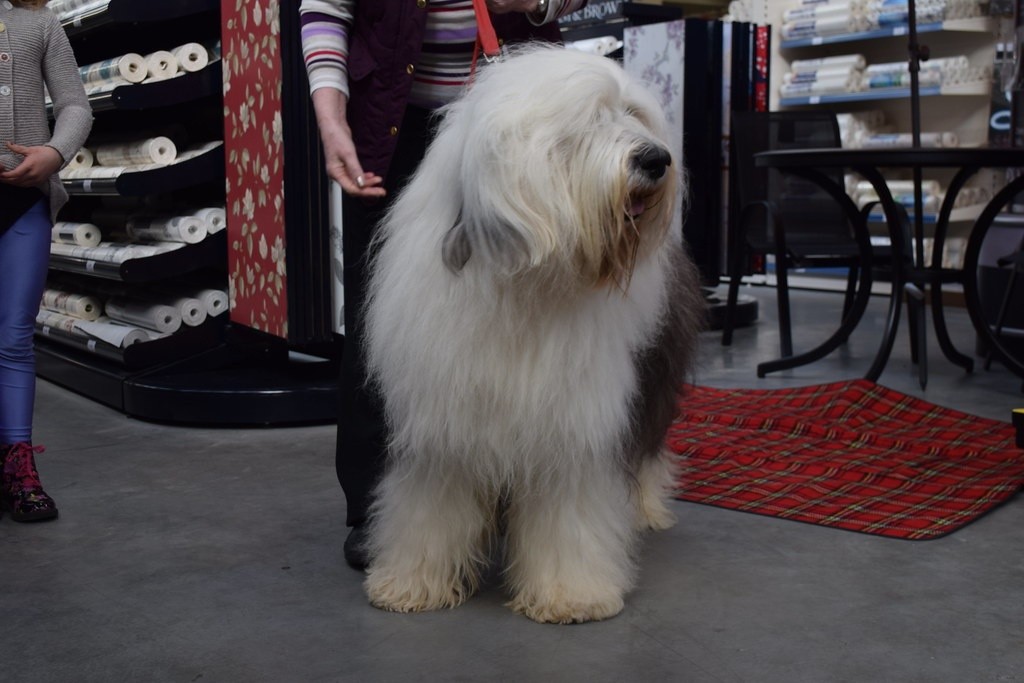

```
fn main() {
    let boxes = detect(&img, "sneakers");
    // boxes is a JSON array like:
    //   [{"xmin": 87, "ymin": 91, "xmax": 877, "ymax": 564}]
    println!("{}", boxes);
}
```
[{"xmin": 0, "ymin": 440, "xmax": 58, "ymax": 523}]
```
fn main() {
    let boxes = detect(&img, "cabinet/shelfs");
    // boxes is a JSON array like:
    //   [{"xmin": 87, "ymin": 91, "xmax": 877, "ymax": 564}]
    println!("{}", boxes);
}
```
[{"xmin": 764, "ymin": 12, "xmax": 996, "ymax": 289}]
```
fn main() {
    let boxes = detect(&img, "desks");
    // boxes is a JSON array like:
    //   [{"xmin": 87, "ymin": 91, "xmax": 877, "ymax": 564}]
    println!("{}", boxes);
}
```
[{"xmin": 757, "ymin": 144, "xmax": 1024, "ymax": 393}]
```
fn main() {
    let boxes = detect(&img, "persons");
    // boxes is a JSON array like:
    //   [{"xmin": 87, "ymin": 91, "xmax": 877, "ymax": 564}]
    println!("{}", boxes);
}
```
[
  {"xmin": 297, "ymin": 0, "xmax": 583, "ymax": 574},
  {"xmin": 0, "ymin": 0, "xmax": 94, "ymax": 524}
]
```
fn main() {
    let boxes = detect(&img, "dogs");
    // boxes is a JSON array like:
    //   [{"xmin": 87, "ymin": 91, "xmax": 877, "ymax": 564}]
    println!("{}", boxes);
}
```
[{"xmin": 360, "ymin": 42, "xmax": 707, "ymax": 624}]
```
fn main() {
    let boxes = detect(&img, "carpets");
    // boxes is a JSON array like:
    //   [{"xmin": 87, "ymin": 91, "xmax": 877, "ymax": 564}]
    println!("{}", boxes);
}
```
[{"xmin": 658, "ymin": 379, "xmax": 1024, "ymax": 541}]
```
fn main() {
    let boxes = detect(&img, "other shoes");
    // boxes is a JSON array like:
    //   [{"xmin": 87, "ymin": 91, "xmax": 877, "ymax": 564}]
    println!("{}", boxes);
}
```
[{"xmin": 344, "ymin": 526, "xmax": 374, "ymax": 570}]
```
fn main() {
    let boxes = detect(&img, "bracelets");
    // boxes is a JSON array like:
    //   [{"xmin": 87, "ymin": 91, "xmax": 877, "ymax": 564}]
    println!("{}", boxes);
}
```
[{"xmin": 537, "ymin": 0, "xmax": 547, "ymax": 13}]
